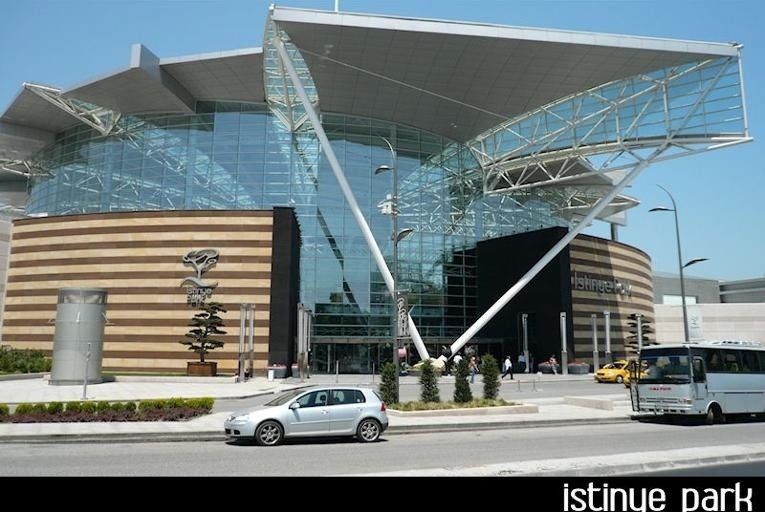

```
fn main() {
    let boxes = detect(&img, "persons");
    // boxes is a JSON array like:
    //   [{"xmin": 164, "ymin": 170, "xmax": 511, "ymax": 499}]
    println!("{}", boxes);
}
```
[
  {"xmin": 468, "ymin": 356, "xmax": 479, "ymax": 384},
  {"xmin": 502, "ymin": 355, "xmax": 513, "ymax": 379},
  {"xmin": 549, "ymin": 354, "xmax": 558, "ymax": 374},
  {"xmin": 529, "ymin": 352, "xmax": 534, "ymax": 372},
  {"xmin": 453, "ymin": 352, "xmax": 462, "ymax": 366},
  {"xmin": 643, "ymin": 359, "xmax": 660, "ymax": 377},
  {"xmin": 518, "ymin": 352, "xmax": 526, "ymax": 372}
]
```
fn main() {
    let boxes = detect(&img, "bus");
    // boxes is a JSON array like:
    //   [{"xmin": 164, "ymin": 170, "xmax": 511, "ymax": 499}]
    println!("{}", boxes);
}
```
[{"xmin": 628, "ymin": 340, "xmax": 765, "ymax": 425}]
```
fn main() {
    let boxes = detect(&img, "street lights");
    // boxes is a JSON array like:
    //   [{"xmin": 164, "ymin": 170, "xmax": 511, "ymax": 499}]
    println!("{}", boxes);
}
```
[
  {"xmin": 560, "ymin": 312, "xmax": 569, "ymax": 374},
  {"xmin": 373, "ymin": 137, "xmax": 413, "ymax": 403},
  {"xmin": 648, "ymin": 183, "xmax": 709, "ymax": 342},
  {"xmin": 240, "ymin": 303, "xmax": 255, "ymax": 381},
  {"xmin": 297, "ymin": 303, "xmax": 312, "ymax": 378},
  {"xmin": 591, "ymin": 313, "xmax": 600, "ymax": 370},
  {"xmin": 604, "ymin": 310, "xmax": 612, "ymax": 362},
  {"xmin": 522, "ymin": 313, "xmax": 530, "ymax": 372},
  {"xmin": 636, "ymin": 312, "xmax": 643, "ymax": 352}
]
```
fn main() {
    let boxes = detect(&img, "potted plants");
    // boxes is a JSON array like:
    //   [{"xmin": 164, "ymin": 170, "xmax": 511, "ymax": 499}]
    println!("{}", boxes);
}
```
[{"xmin": 179, "ymin": 302, "xmax": 226, "ymax": 377}]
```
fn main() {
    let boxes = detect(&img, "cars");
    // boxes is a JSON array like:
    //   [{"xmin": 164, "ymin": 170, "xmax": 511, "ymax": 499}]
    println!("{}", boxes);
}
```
[
  {"xmin": 224, "ymin": 384, "xmax": 388, "ymax": 446},
  {"xmin": 594, "ymin": 358, "xmax": 648, "ymax": 388}
]
